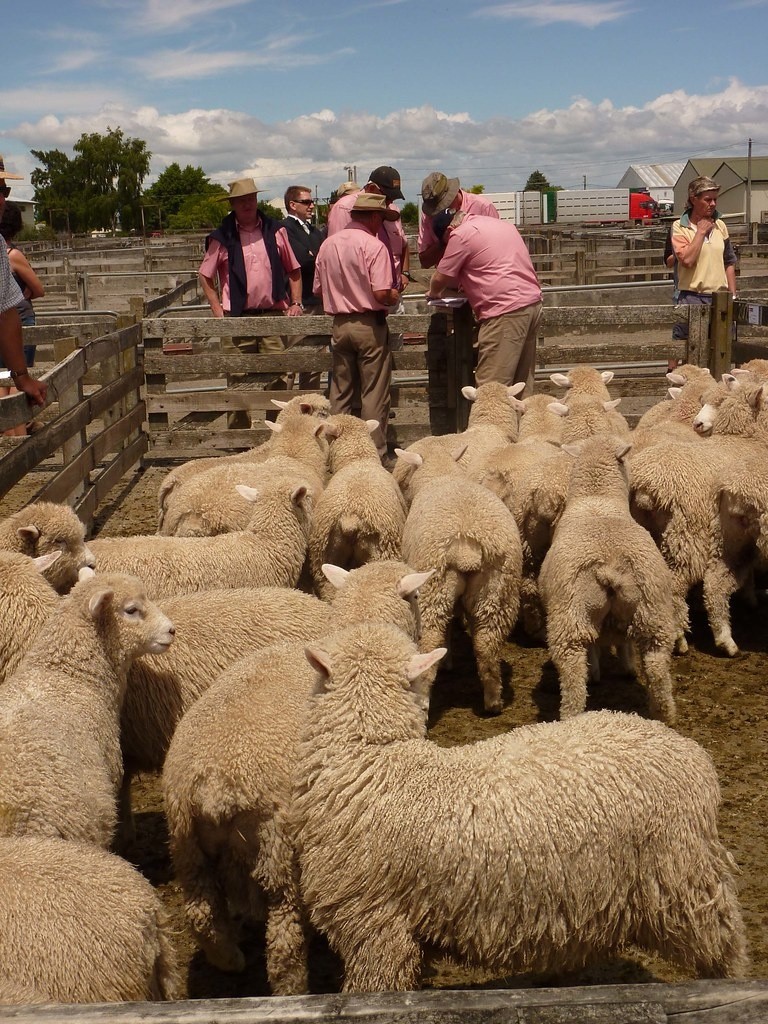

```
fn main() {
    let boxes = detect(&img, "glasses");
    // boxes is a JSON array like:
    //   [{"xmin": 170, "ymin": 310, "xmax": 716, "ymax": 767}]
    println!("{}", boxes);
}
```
[
  {"xmin": 293, "ymin": 199, "xmax": 314, "ymax": 204},
  {"xmin": 0, "ymin": 186, "xmax": 11, "ymax": 198}
]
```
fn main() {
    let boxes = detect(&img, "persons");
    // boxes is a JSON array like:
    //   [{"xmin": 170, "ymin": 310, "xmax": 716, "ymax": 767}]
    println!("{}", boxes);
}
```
[
  {"xmin": 663, "ymin": 175, "xmax": 738, "ymax": 373},
  {"xmin": 198, "ymin": 165, "xmax": 544, "ymax": 472},
  {"xmin": 0, "ymin": 153, "xmax": 51, "ymax": 437}
]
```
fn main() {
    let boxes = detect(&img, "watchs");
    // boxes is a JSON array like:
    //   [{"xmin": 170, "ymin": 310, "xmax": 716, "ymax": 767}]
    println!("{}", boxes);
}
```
[
  {"xmin": 732, "ymin": 296, "xmax": 738, "ymax": 299},
  {"xmin": 401, "ymin": 271, "xmax": 410, "ymax": 277}
]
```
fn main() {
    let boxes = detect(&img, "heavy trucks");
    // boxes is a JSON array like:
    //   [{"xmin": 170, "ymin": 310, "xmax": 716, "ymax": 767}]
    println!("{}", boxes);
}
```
[{"xmin": 417, "ymin": 187, "xmax": 660, "ymax": 226}]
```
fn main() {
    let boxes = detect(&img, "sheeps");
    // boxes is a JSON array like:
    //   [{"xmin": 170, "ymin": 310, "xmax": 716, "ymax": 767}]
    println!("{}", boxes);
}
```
[
  {"xmin": 81, "ymin": 483, "xmax": 311, "ymax": 601},
  {"xmin": 1, "ymin": 834, "xmax": 185, "ymax": 1008},
  {"xmin": 287, "ymin": 621, "xmax": 753, "ymax": 996},
  {"xmin": 0, "ymin": 502, "xmax": 97, "ymax": 594},
  {"xmin": 150, "ymin": 358, "xmax": 768, "ymax": 729},
  {"xmin": 160, "ymin": 559, "xmax": 440, "ymax": 998},
  {"xmin": 1, "ymin": 567, "xmax": 177, "ymax": 850},
  {"xmin": 117, "ymin": 584, "xmax": 334, "ymax": 767}
]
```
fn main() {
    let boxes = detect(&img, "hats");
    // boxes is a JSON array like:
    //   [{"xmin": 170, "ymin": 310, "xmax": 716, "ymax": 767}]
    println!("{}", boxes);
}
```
[
  {"xmin": 433, "ymin": 207, "xmax": 458, "ymax": 251},
  {"xmin": 421, "ymin": 172, "xmax": 460, "ymax": 216},
  {"xmin": 351, "ymin": 193, "xmax": 400, "ymax": 222},
  {"xmin": 369, "ymin": 166, "xmax": 405, "ymax": 201},
  {"xmin": 338, "ymin": 181, "xmax": 361, "ymax": 196},
  {"xmin": 215, "ymin": 177, "xmax": 267, "ymax": 202},
  {"xmin": 0, "ymin": 154, "xmax": 25, "ymax": 181}
]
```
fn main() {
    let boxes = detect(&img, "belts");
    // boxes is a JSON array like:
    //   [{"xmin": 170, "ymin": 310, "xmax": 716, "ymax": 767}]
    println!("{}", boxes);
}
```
[{"xmin": 245, "ymin": 309, "xmax": 272, "ymax": 315}]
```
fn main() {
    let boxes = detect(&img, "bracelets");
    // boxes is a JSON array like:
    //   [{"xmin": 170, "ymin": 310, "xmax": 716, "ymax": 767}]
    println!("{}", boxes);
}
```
[
  {"xmin": 10, "ymin": 369, "xmax": 28, "ymax": 378},
  {"xmin": 292, "ymin": 303, "xmax": 304, "ymax": 309}
]
